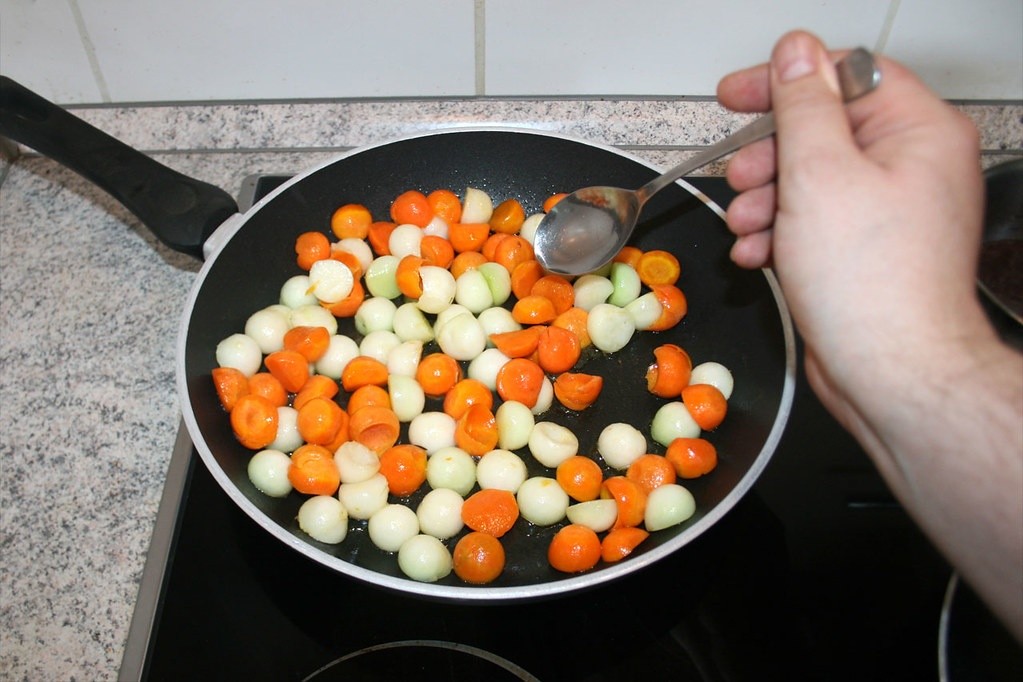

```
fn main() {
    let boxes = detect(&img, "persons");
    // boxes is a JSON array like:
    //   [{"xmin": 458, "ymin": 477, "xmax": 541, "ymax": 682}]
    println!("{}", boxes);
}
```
[{"xmin": 715, "ymin": 30, "xmax": 1023, "ymax": 640}]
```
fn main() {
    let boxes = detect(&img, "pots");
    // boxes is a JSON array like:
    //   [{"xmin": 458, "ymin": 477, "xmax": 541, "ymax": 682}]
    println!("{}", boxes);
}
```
[{"xmin": 1, "ymin": 72, "xmax": 797, "ymax": 601}]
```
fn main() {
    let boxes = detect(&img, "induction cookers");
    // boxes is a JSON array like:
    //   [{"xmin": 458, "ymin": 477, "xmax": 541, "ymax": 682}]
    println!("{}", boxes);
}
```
[{"xmin": 114, "ymin": 162, "xmax": 1022, "ymax": 682}]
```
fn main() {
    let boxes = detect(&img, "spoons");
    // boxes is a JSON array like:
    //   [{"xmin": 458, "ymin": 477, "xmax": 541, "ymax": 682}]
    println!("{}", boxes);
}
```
[{"xmin": 534, "ymin": 42, "xmax": 881, "ymax": 275}]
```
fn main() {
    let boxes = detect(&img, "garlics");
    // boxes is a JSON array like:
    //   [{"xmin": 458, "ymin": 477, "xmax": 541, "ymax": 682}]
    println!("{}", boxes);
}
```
[{"xmin": 207, "ymin": 156, "xmax": 740, "ymax": 586}]
